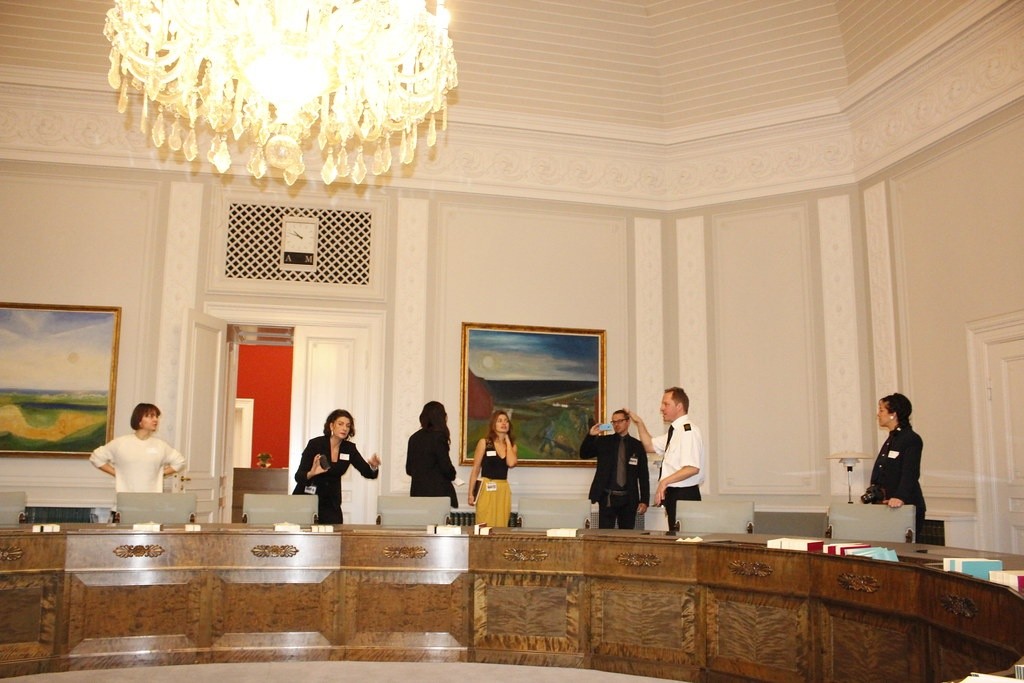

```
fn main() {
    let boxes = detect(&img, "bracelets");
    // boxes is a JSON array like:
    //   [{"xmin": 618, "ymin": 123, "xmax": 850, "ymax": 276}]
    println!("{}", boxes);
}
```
[
  {"xmin": 468, "ymin": 496, "xmax": 474, "ymax": 498},
  {"xmin": 370, "ymin": 465, "xmax": 378, "ymax": 472}
]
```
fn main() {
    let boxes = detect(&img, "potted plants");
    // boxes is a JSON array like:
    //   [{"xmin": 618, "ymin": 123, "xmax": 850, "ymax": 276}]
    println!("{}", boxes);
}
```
[{"xmin": 257, "ymin": 452, "xmax": 272, "ymax": 469}]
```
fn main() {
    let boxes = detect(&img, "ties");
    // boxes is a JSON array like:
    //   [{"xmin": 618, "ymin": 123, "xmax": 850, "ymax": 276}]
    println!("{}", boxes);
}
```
[
  {"xmin": 616, "ymin": 437, "xmax": 627, "ymax": 487},
  {"xmin": 658, "ymin": 425, "xmax": 674, "ymax": 481}
]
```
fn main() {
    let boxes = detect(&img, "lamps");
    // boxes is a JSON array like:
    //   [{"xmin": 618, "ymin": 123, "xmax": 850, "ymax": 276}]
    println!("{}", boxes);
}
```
[
  {"xmin": 827, "ymin": 450, "xmax": 874, "ymax": 504},
  {"xmin": 100, "ymin": 0, "xmax": 457, "ymax": 189}
]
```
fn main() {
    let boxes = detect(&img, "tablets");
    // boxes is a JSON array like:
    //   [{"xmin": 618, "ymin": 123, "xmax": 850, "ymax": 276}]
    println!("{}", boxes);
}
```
[{"xmin": 473, "ymin": 479, "xmax": 483, "ymax": 504}]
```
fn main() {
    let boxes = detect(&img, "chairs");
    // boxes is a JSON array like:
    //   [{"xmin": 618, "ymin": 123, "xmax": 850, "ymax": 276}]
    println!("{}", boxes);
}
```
[
  {"xmin": 583, "ymin": 510, "xmax": 647, "ymax": 530},
  {"xmin": 115, "ymin": 492, "xmax": 199, "ymax": 522},
  {"xmin": 242, "ymin": 494, "xmax": 319, "ymax": 523},
  {"xmin": 674, "ymin": 499, "xmax": 755, "ymax": 533},
  {"xmin": 376, "ymin": 496, "xmax": 451, "ymax": 525},
  {"xmin": 826, "ymin": 503, "xmax": 916, "ymax": 542},
  {"xmin": 18, "ymin": 506, "xmax": 98, "ymax": 523}
]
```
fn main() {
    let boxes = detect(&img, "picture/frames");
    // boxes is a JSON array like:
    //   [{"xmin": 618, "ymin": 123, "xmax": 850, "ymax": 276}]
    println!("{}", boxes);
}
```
[
  {"xmin": 459, "ymin": 321, "xmax": 608, "ymax": 467},
  {"xmin": 0, "ymin": 302, "xmax": 123, "ymax": 458}
]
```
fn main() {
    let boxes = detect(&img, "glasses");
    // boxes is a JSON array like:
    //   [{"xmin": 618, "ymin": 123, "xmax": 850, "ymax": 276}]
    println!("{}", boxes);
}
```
[{"xmin": 610, "ymin": 418, "xmax": 627, "ymax": 425}]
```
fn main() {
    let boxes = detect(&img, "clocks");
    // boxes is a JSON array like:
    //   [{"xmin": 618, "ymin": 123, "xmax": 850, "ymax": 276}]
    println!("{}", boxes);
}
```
[{"xmin": 279, "ymin": 214, "xmax": 320, "ymax": 273}]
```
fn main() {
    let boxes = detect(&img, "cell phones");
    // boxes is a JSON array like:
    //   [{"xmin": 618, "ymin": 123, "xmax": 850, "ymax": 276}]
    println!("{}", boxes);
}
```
[{"xmin": 599, "ymin": 424, "xmax": 612, "ymax": 431}]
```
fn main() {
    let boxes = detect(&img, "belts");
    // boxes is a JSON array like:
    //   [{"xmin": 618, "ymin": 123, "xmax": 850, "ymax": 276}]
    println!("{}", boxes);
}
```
[{"xmin": 604, "ymin": 488, "xmax": 629, "ymax": 508}]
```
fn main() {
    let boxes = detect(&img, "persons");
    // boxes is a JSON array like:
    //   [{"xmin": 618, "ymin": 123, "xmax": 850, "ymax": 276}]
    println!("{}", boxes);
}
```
[
  {"xmin": 406, "ymin": 401, "xmax": 458, "ymax": 509},
  {"xmin": 579, "ymin": 409, "xmax": 650, "ymax": 530},
  {"xmin": 88, "ymin": 402, "xmax": 185, "ymax": 522},
  {"xmin": 870, "ymin": 392, "xmax": 927, "ymax": 544},
  {"xmin": 623, "ymin": 387, "xmax": 705, "ymax": 532},
  {"xmin": 468, "ymin": 410, "xmax": 517, "ymax": 529},
  {"xmin": 292, "ymin": 409, "xmax": 382, "ymax": 524}
]
